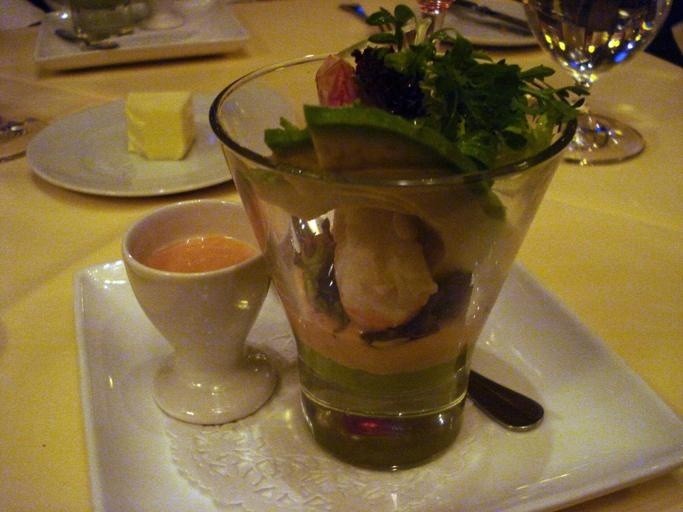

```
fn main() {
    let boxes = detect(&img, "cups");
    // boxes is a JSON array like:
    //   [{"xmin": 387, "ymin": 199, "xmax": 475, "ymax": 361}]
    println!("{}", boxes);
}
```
[{"xmin": 208, "ymin": 47, "xmax": 578, "ymax": 472}]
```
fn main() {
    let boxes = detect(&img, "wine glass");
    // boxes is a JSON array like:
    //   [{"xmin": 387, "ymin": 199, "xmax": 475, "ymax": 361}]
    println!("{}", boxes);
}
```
[
  {"xmin": 520, "ymin": 0, "xmax": 672, "ymax": 165},
  {"xmin": 120, "ymin": 198, "xmax": 279, "ymax": 424}
]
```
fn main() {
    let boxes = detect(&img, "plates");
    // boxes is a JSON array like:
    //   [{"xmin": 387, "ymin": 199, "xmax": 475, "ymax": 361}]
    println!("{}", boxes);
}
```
[
  {"xmin": 34, "ymin": 11, "xmax": 248, "ymax": 71},
  {"xmin": 74, "ymin": 255, "xmax": 683, "ymax": 512},
  {"xmin": 26, "ymin": 102, "xmax": 290, "ymax": 198}
]
